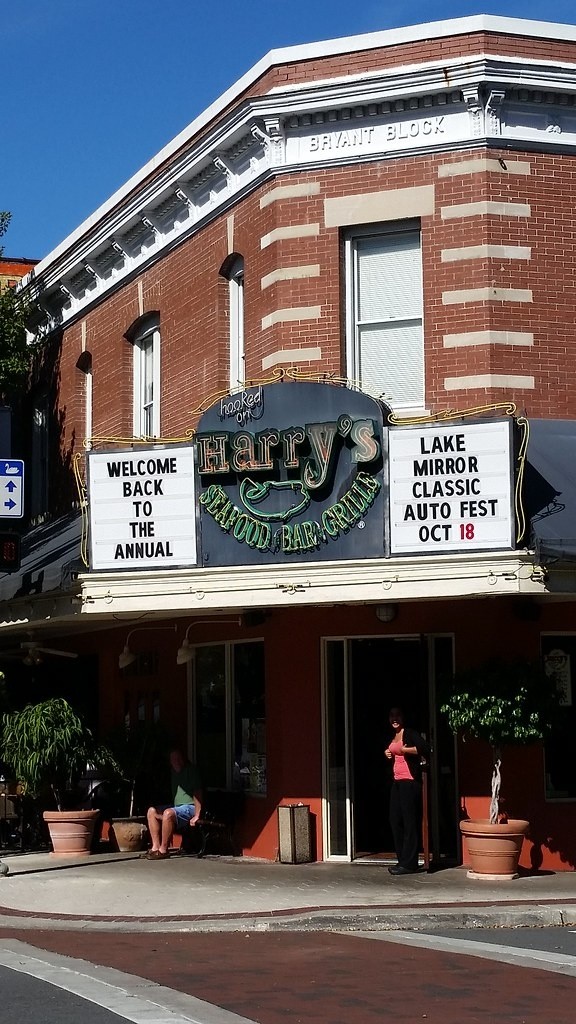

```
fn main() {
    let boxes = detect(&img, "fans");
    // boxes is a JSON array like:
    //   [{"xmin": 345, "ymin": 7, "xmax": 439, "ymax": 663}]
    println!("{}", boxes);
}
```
[{"xmin": 1, "ymin": 630, "xmax": 78, "ymax": 659}]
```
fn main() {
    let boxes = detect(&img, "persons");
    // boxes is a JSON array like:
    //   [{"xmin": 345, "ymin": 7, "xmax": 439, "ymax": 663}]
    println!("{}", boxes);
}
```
[
  {"xmin": 139, "ymin": 745, "xmax": 209, "ymax": 862},
  {"xmin": 381, "ymin": 702, "xmax": 431, "ymax": 876}
]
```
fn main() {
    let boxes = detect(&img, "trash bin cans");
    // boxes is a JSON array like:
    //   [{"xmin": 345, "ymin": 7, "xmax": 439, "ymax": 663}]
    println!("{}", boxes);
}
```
[{"xmin": 277, "ymin": 803, "xmax": 312, "ymax": 865}]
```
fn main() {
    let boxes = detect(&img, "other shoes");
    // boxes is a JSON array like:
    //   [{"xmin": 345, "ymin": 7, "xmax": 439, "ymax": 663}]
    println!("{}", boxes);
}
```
[
  {"xmin": 139, "ymin": 850, "xmax": 152, "ymax": 859},
  {"xmin": 147, "ymin": 851, "xmax": 170, "ymax": 860},
  {"xmin": 388, "ymin": 865, "xmax": 418, "ymax": 875}
]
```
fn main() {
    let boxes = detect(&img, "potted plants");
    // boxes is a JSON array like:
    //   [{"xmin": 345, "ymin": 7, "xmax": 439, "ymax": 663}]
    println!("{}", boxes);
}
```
[
  {"xmin": 99, "ymin": 719, "xmax": 178, "ymax": 852},
  {"xmin": 439, "ymin": 650, "xmax": 567, "ymax": 874},
  {"xmin": 0, "ymin": 697, "xmax": 125, "ymax": 855}
]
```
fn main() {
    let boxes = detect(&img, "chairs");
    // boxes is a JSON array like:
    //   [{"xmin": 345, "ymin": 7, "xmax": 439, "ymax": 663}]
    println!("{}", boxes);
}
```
[{"xmin": 174, "ymin": 790, "xmax": 246, "ymax": 858}]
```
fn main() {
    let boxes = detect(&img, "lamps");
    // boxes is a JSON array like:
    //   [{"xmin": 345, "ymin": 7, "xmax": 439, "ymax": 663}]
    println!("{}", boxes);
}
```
[
  {"xmin": 177, "ymin": 616, "xmax": 241, "ymax": 665},
  {"xmin": 375, "ymin": 604, "xmax": 397, "ymax": 623},
  {"xmin": 23, "ymin": 651, "xmax": 43, "ymax": 665},
  {"xmin": 119, "ymin": 623, "xmax": 178, "ymax": 669}
]
